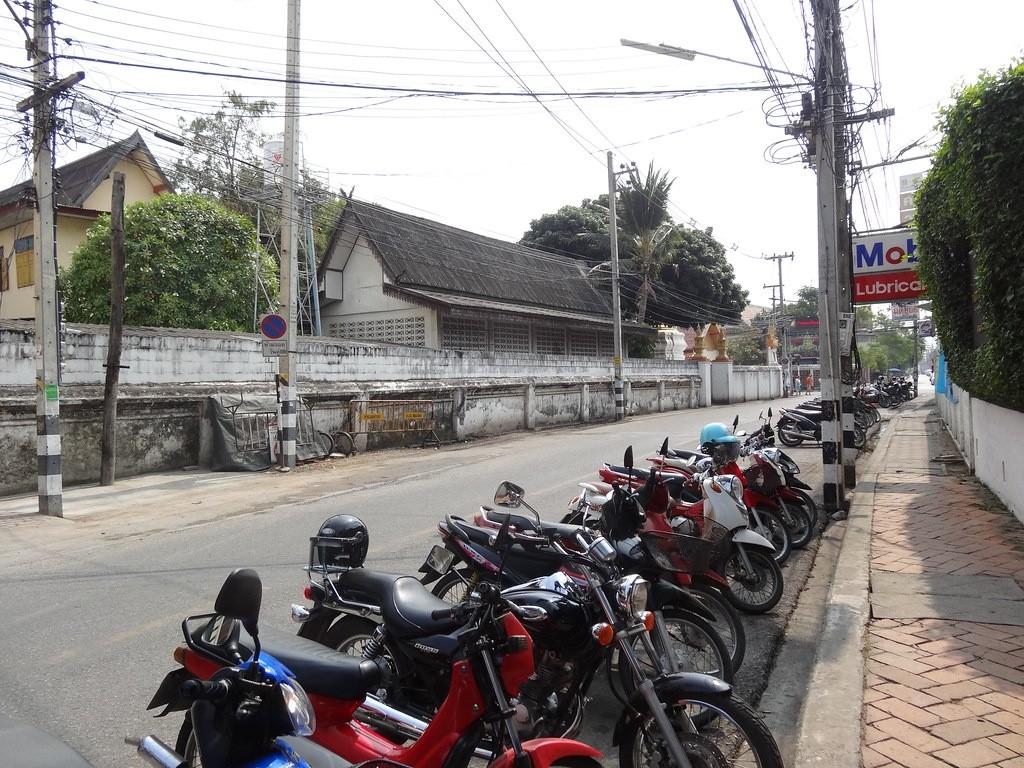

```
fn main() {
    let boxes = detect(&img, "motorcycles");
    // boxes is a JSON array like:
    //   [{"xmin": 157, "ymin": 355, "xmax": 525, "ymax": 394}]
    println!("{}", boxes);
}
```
[
  {"xmin": 290, "ymin": 481, "xmax": 782, "ymax": 767},
  {"xmin": 417, "ymin": 375, "xmax": 918, "ymax": 730},
  {"xmin": 121, "ymin": 514, "xmax": 606, "ymax": 768}
]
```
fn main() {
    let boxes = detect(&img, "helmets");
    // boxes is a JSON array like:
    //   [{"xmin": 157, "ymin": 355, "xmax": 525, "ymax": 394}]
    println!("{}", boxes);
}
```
[
  {"xmin": 700, "ymin": 422, "xmax": 739, "ymax": 448},
  {"xmin": 317, "ymin": 514, "xmax": 369, "ymax": 568}
]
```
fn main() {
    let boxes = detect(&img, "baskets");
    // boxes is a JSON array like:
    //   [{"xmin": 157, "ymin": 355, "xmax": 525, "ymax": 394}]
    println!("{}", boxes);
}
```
[
  {"xmin": 742, "ymin": 462, "xmax": 782, "ymax": 493},
  {"xmin": 673, "ymin": 515, "xmax": 730, "ymax": 563},
  {"xmin": 640, "ymin": 529, "xmax": 717, "ymax": 575}
]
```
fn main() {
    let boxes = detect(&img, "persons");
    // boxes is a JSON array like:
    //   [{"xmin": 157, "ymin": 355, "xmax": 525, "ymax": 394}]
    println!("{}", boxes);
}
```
[
  {"xmin": 795, "ymin": 376, "xmax": 801, "ymax": 396},
  {"xmin": 805, "ymin": 374, "xmax": 813, "ymax": 395}
]
